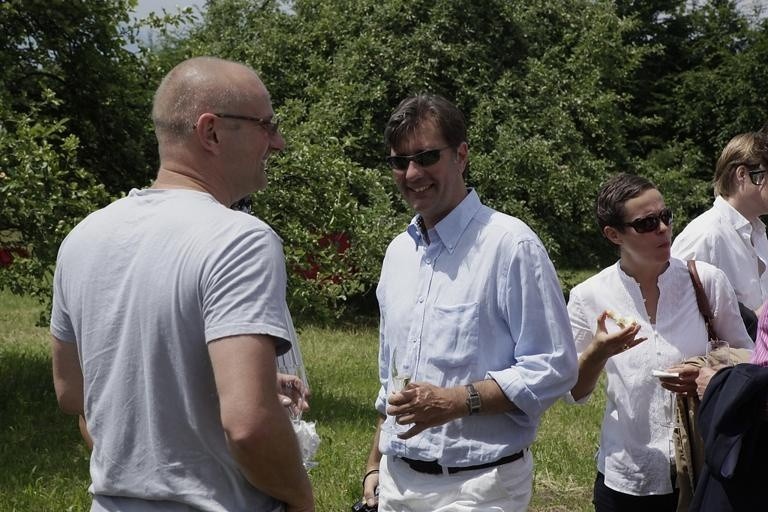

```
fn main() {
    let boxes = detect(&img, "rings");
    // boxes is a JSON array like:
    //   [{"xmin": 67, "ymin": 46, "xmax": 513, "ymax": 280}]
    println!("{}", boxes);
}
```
[{"xmin": 624, "ymin": 344, "xmax": 629, "ymax": 350}]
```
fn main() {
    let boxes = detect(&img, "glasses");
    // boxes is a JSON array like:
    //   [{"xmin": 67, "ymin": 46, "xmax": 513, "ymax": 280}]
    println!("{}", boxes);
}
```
[
  {"xmin": 609, "ymin": 207, "xmax": 674, "ymax": 233},
  {"xmin": 385, "ymin": 141, "xmax": 460, "ymax": 171},
  {"xmin": 192, "ymin": 113, "xmax": 281, "ymax": 137},
  {"xmin": 748, "ymin": 169, "xmax": 768, "ymax": 185}
]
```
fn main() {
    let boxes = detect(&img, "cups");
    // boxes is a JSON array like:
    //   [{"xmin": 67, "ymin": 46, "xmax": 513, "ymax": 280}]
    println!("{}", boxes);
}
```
[{"xmin": 704, "ymin": 340, "xmax": 729, "ymax": 370}]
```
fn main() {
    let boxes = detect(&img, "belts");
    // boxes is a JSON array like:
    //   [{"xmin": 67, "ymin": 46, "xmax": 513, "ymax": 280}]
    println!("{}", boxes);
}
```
[{"xmin": 400, "ymin": 447, "xmax": 530, "ymax": 475}]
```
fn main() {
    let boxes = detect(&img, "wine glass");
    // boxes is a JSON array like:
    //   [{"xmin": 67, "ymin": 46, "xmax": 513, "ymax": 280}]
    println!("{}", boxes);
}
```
[
  {"xmin": 377, "ymin": 342, "xmax": 418, "ymax": 437},
  {"xmin": 278, "ymin": 365, "xmax": 317, "ymax": 468}
]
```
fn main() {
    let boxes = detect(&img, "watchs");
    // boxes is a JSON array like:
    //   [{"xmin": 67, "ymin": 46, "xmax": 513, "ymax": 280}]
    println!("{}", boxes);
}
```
[{"xmin": 464, "ymin": 384, "xmax": 481, "ymax": 416}]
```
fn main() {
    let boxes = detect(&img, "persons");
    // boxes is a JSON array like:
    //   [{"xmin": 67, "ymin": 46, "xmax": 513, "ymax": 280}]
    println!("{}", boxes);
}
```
[
  {"xmin": 48, "ymin": 56, "xmax": 315, "ymax": 511},
  {"xmin": 566, "ymin": 172, "xmax": 750, "ymax": 512},
  {"xmin": 361, "ymin": 94, "xmax": 580, "ymax": 512},
  {"xmin": 670, "ymin": 132, "xmax": 768, "ymax": 408}
]
